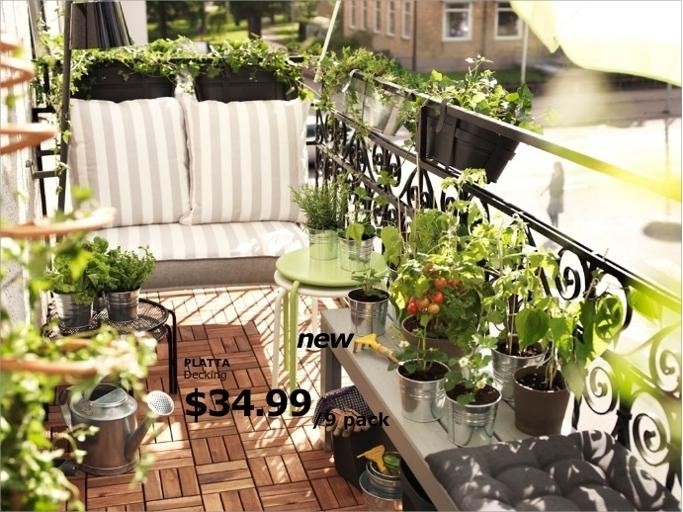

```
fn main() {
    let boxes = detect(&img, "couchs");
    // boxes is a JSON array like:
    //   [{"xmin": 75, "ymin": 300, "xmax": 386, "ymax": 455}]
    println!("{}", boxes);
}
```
[{"xmin": 34, "ymin": 91, "xmax": 312, "ymax": 291}]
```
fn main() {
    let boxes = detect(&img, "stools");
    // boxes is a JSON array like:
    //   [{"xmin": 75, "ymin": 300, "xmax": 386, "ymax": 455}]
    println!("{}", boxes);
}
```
[
  {"xmin": 39, "ymin": 297, "xmax": 179, "ymax": 395},
  {"xmin": 271, "ymin": 242, "xmax": 390, "ymax": 417}
]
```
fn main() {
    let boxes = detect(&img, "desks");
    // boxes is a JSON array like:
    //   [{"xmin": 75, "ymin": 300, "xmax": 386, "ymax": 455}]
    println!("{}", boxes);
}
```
[{"xmin": 321, "ymin": 302, "xmax": 608, "ymax": 511}]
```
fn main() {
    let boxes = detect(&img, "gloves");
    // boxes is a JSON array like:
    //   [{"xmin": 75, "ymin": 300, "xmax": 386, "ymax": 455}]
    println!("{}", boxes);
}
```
[
  {"xmin": 337, "ymin": 409, "xmax": 370, "ymax": 431},
  {"xmin": 321, "ymin": 408, "xmax": 351, "ymax": 437}
]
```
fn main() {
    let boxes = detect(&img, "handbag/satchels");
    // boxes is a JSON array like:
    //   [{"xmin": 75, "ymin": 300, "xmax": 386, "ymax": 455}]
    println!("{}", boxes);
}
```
[{"xmin": 312, "ymin": 385, "xmax": 373, "ymax": 425}]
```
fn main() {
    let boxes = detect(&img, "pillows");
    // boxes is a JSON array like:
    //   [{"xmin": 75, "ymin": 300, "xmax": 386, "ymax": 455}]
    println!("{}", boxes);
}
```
[
  {"xmin": 49, "ymin": 94, "xmax": 191, "ymax": 228},
  {"xmin": 179, "ymin": 97, "xmax": 312, "ymax": 225}
]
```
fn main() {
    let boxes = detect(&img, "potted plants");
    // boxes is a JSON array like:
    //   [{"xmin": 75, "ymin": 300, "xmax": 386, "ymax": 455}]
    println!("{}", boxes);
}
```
[
  {"xmin": 161, "ymin": 33, "xmax": 315, "ymax": 101},
  {"xmin": 40, "ymin": 230, "xmax": 158, "ymax": 327},
  {"xmin": 418, "ymin": 52, "xmax": 560, "ymax": 186},
  {"xmin": 315, "ymin": 44, "xmax": 424, "ymax": 137},
  {"xmin": 35, "ymin": 33, "xmax": 180, "ymax": 196},
  {"xmin": 0, "ymin": 404, "xmax": 88, "ymax": 512}
]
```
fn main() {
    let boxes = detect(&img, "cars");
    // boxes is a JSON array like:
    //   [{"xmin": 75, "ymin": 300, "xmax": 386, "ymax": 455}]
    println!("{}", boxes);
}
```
[{"xmin": 307, "ymin": 117, "xmax": 358, "ymax": 165}]
